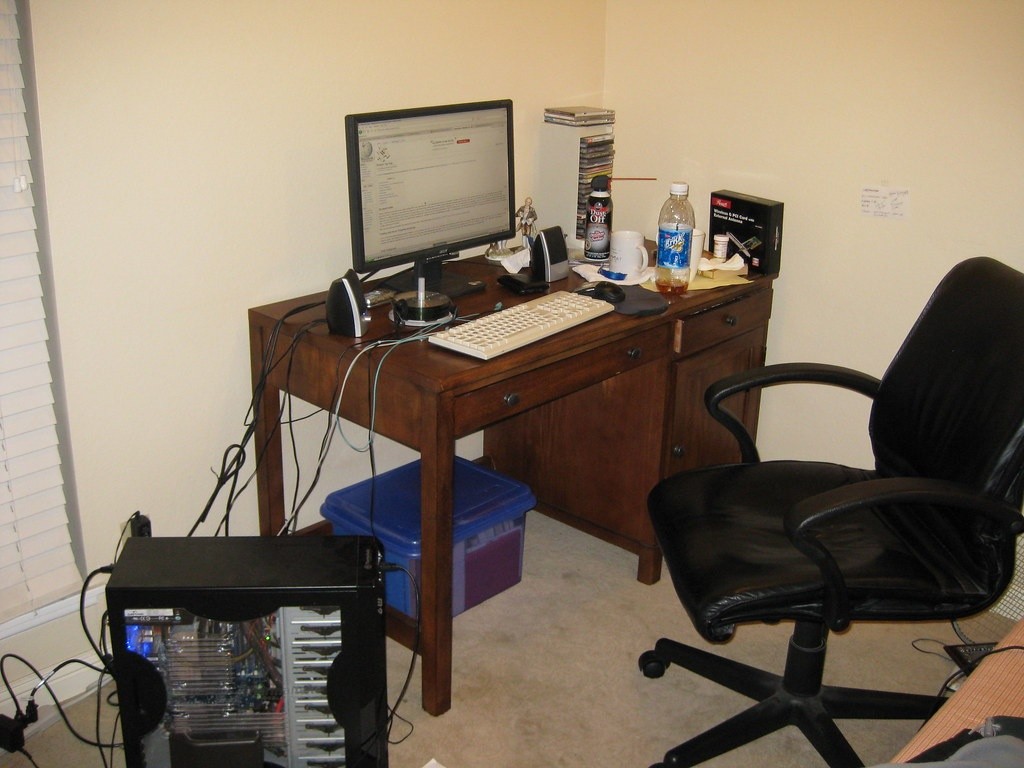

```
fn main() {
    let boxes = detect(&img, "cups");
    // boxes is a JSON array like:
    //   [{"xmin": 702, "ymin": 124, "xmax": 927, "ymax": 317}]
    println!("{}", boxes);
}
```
[
  {"xmin": 610, "ymin": 231, "xmax": 649, "ymax": 281},
  {"xmin": 656, "ymin": 227, "xmax": 705, "ymax": 285}
]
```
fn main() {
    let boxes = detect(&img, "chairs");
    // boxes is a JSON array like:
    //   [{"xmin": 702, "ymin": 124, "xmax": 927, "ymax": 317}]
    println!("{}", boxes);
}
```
[{"xmin": 639, "ymin": 256, "xmax": 1024, "ymax": 768}]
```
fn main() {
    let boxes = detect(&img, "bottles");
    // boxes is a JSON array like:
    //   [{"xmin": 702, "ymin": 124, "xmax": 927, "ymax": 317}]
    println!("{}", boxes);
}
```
[
  {"xmin": 584, "ymin": 175, "xmax": 613, "ymax": 262},
  {"xmin": 653, "ymin": 183, "xmax": 695, "ymax": 295}
]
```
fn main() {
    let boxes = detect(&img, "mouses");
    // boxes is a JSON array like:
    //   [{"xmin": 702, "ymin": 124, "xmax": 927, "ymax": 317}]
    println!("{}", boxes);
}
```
[{"xmin": 572, "ymin": 281, "xmax": 626, "ymax": 303}]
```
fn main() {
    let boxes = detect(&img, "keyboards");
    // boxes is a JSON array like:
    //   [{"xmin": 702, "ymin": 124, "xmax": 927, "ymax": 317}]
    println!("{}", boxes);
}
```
[{"xmin": 428, "ymin": 290, "xmax": 615, "ymax": 360}]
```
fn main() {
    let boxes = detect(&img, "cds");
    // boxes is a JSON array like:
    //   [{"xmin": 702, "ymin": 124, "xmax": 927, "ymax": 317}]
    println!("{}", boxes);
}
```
[{"xmin": 392, "ymin": 291, "xmax": 451, "ymax": 321}]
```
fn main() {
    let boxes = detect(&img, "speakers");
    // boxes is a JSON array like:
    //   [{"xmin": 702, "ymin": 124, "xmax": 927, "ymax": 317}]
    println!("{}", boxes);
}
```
[
  {"xmin": 529, "ymin": 226, "xmax": 568, "ymax": 282},
  {"xmin": 326, "ymin": 269, "xmax": 372, "ymax": 338}
]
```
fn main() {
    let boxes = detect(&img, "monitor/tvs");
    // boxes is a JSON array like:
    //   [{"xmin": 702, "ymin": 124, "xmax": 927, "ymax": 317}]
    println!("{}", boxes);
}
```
[{"xmin": 345, "ymin": 99, "xmax": 517, "ymax": 298}]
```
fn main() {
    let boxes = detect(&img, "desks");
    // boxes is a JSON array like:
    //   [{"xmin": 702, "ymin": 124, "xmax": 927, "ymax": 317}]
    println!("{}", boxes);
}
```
[{"xmin": 247, "ymin": 238, "xmax": 779, "ymax": 718}]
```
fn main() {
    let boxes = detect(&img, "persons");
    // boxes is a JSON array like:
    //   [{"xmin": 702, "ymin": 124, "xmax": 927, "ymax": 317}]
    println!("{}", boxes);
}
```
[{"xmin": 516, "ymin": 196, "xmax": 538, "ymax": 249}]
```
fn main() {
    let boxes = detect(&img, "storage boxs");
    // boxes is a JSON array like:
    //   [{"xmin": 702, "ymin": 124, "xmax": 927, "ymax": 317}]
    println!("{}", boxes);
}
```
[
  {"xmin": 708, "ymin": 189, "xmax": 784, "ymax": 276},
  {"xmin": 319, "ymin": 453, "xmax": 536, "ymax": 619}
]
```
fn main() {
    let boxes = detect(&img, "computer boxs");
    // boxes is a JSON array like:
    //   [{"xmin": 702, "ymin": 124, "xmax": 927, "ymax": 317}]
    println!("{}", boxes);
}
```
[{"xmin": 106, "ymin": 536, "xmax": 390, "ymax": 768}]
